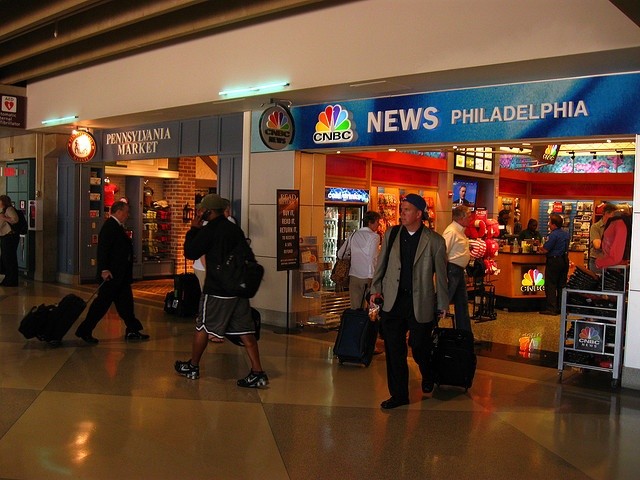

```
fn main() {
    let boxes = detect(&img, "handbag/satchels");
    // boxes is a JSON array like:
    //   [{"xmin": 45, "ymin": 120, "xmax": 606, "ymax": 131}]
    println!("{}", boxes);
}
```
[
  {"xmin": 330, "ymin": 257, "xmax": 350, "ymax": 286},
  {"xmin": 224, "ymin": 307, "xmax": 261, "ymax": 347},
  {"xmin": 163, "ymin": 292, "xmax": 174, "ymax": 314},
  {"xmin": 18, "ymin": 304, "xmax": 54, "ymax": 339}
]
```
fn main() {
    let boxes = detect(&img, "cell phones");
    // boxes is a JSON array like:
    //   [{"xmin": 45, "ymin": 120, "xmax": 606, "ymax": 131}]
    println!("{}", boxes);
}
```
[{"xmin": 201, "ymin": 210, "xmax": 210, "ymax": 219}]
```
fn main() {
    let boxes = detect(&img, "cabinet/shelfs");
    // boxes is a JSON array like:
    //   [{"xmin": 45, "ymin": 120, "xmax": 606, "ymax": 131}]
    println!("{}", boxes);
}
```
[
  {"xmin": 323, "ymin": 175, "xmax": 372, "ymax": 291},
  {"xmin": 372, "ymin": 180, "xmax": 440, "ymax": 252},
  {"xmin": 546, "ymin": 201, "xmax": 571, "ymax": 250},
  {"xmin": 571, "ymin": 201, "xmax": 594, "ymax": 258}
]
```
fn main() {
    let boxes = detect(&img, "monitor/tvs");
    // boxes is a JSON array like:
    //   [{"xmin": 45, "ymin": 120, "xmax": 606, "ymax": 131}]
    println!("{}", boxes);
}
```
[{"xmin": 453, "ymin": 180, "xmax": 479, "ymax": 212}]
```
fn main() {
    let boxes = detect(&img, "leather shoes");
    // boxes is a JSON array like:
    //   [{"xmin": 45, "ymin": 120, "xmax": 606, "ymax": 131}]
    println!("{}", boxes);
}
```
[
  {"xmin": 380, "ymin": 395, "xmax": 410, "ymax": 409},
  {"xmin": 125, "ymin": 330, "xmax": 151, "ymax": 342},
  {"xmin": 74, "ymin": 326, "xmax": 99, "ymax": 345},
  {"xmin": 421, "ymin": 377, "xmax": 434, "ymax": 393}
]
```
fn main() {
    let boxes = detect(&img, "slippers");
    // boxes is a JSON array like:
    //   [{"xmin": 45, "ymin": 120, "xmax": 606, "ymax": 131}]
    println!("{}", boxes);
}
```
[{"xmin": 208, "ymin": 334, "xmax": 224, "ymax": 344}]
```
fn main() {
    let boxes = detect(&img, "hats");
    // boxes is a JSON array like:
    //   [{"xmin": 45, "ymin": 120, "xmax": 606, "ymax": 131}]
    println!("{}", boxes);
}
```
[
  {"xmin": 195, "ymin": 193, "xmax": 226, "ymax": 211},
  {"xmin": 403, "ymin": 194, "xmax": 428, "ymax": 221}
]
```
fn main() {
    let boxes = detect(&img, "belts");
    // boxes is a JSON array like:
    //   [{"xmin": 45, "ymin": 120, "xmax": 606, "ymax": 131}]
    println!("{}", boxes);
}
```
[{"xmin": 399, "ymin": 288, "xmax": 413, "ymax": 295}]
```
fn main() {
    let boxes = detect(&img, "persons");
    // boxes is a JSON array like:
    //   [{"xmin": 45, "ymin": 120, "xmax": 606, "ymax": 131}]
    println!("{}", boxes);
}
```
[
  {"xmin": 1, "ymin": 193, "xmax": 21, "ymax": 285},
  {"xmin": 370, "ymin": 194, "xmax": 450, "ymax": 411},
  {"xmin": 588, "ymin": 204, "xmax": 618, "ymax": 272},
  {"xmin": 337, "ymin": 210, "xmax": 380, "ymax": 309},
  {"xmin": 173, "ymin": 194, "xmax": 269, "ymax": 389},
  {"xmin": 441, "ymin": 205, "xmax": 473, "ymax": 332},
  {"xmin": 192, "ymin": 198, "xmax": 236, "ymax": 344},
  {"xmin": 74, "ymin": 200, "xmax": 151, "ymax": 345},
  {"xmin": 520, "ymin": 219, "xmax": 542, "ymax": 245},
  {"xmin": 539, "ymin": 211, "xmax": 570, "ymax": 315},
  {"xmin": 498, "ymin": 209, "xmax": 510, "ymax": 238},
  {"xmin": 454, "ymin": 185, "xmax": 471, "ymax": 204}
]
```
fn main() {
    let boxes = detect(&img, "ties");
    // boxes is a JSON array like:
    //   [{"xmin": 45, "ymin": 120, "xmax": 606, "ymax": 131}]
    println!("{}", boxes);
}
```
[{"xmin": 460, "ymin": 201, "xmax": 462, "ymax": 204}]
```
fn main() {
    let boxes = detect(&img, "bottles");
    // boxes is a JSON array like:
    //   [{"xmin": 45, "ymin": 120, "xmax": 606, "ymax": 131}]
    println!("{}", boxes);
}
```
[
  {"xmin": 324, "ymin": 257, "xmax": 336, "ymax": 273},
  {"xmin": 324, "ymin": 239, "xmax": 336, "ymax": 255},
  {"xmin": 531, "ymin": 238, "xmax": 537, "ymax": 254},
  {"xmin": 322, "ymin": 272, "xmax": 335, "ymax": 287},
  {"xmin": 325, "ymin": 207, "xmax": 338, "ymax": 219},
  {"xmin": 341, "ymin": 223, "xmax": 357, "ymax": 240},
  {"xmin": 513, "ymin": 238, "xmax": 520, "ymax": 253},
  {"xmin": 343, "ymin": 207, "xmax": 357, "ymax": 219},
  {"xmin": 325, "ymin": 221, "xmax": 336, "ymax": 238}
]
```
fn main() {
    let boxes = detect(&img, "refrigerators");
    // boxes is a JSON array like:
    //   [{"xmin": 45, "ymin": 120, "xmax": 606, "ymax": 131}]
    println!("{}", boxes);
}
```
[{"xmin": 321, "ymin": 186, "xmax": 375, "ymax": 314}]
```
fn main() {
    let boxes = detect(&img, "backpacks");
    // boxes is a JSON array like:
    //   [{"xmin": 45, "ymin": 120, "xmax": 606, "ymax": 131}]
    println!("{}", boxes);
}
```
[
  {"xmin": 2, "ymin": 206, "xmax": 28, "ymax": 235},
  {"xmin": 211, "ymin": 222, "xmax": 264, "ymax": 298}
]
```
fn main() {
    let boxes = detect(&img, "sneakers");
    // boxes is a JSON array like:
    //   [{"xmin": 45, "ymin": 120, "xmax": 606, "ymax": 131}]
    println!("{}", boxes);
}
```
[
  {"xmin": 237, "ymin": 367, "xmax": 270, "ymax": 388},
  {"xmin": 539, "ymin": 309, "xmax": 557, "ymax": 316},
  {"xmin": 174, "ymin": 358, "xmax": 200, "ymax": 380},
  {"xmin": 1, "ymin": 281, "xmax": 18, "ymax": 286}
]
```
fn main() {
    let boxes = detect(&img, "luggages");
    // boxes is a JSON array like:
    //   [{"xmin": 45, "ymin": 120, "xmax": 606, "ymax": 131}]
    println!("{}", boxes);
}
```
[
  {"xmin": 173, "ymin": 255, "xmax": 200, "ymax": 321},
  {"xmin": 39, "ymin": 276, "xmax": 110, "ymax": 348},
  {"xmin": 332, "ymin": 283, "xmax": 379, "ymax": 368},
  {"xmin": 430, "ymin": 308, "xmax": 477, "ymax": 392}
]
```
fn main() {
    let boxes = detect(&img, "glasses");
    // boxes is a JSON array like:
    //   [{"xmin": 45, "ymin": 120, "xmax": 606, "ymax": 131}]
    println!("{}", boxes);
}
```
[{"xmin": 503, "ymin": 204, "xmax": 511, "ymax": 206}]
